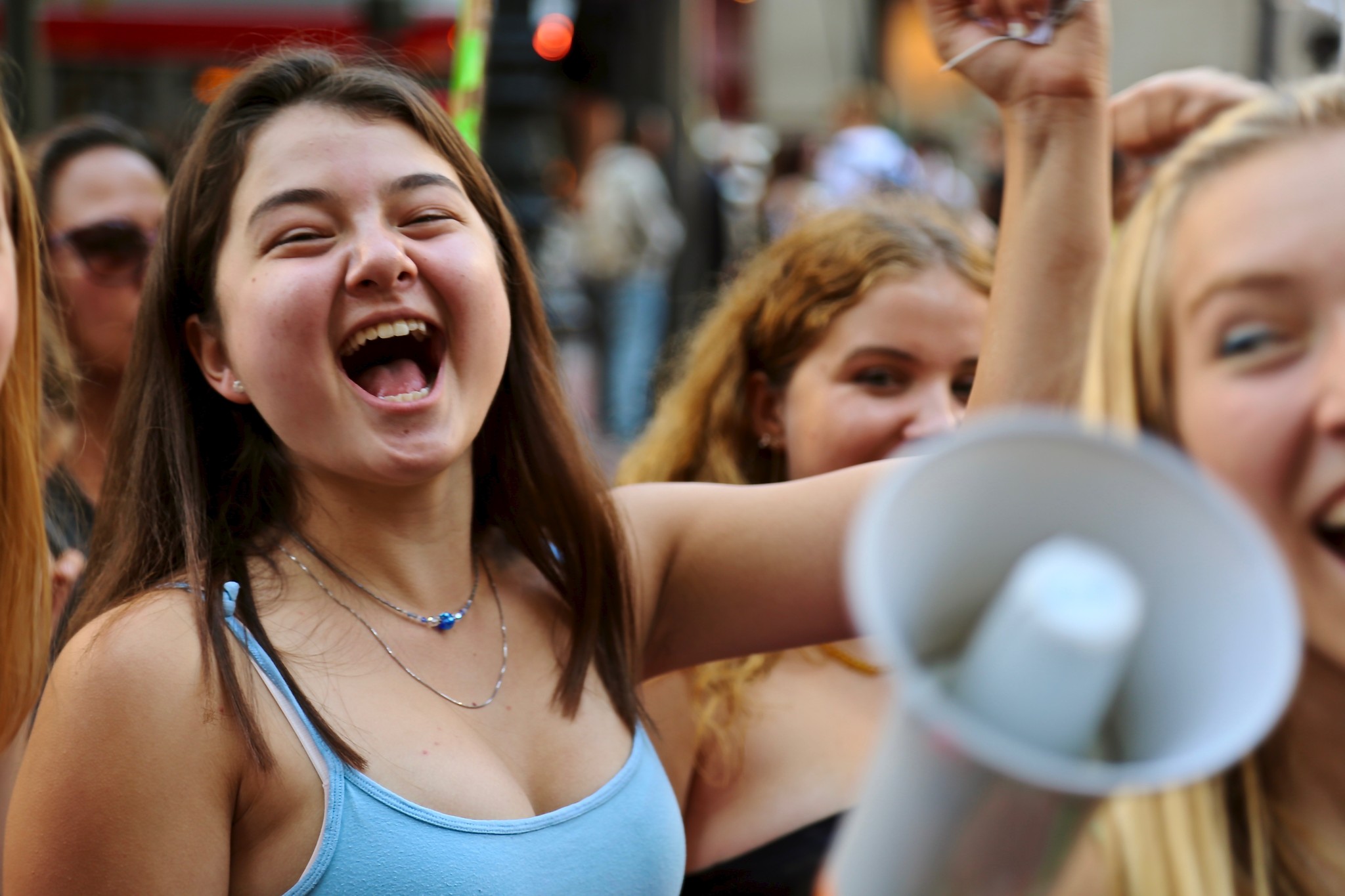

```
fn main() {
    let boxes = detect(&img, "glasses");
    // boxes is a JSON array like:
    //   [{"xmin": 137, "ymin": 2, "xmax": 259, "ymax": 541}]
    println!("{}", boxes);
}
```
[{"xmin": 43, "ymin": 219, "xmax": 151, "ymax": 283}]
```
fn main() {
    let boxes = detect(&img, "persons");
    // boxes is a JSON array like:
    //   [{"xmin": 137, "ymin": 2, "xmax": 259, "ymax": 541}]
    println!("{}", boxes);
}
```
[{"xmin": 0, "ymin": 0, "xmax": 1345, "ymax": 896}]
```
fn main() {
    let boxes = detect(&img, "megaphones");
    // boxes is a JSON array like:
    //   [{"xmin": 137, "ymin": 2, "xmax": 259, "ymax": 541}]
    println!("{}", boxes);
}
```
[{"xmin": 807, "ymin": 417, "xmax": 1305, "ymax": 895}]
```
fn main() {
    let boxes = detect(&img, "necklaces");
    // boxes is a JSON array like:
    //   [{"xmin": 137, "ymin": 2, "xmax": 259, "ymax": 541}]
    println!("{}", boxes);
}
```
[
  {"xmin": 285, "ymin": 525, "xmax": 481, "ymax": 627},
  {"xmin": 262, "ymin": 531, "xmax": 509, "ymax": 710}
]
